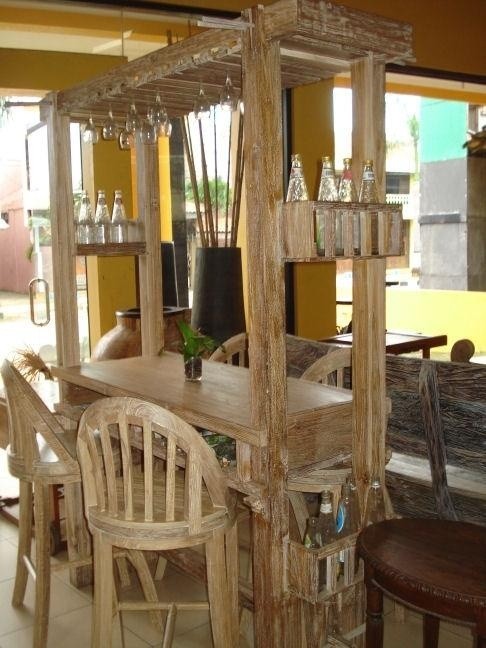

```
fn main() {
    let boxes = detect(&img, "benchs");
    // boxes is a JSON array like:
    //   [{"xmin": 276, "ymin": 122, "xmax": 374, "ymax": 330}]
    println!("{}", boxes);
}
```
[{"xmin": 282, "ymin": 329, "xmax": 486, "ymax": 531}]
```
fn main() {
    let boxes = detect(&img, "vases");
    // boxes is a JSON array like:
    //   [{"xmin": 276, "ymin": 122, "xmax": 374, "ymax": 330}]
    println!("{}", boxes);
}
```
[{"xmin": 87, "ymin": 305, "xmax": 214, "ymax": 366}]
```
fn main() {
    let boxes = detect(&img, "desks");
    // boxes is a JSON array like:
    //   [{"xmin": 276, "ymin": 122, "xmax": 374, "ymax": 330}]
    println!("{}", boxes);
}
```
[{"xmin": 319, "ymin": 325, "xmax": 450, "ymax": 362}]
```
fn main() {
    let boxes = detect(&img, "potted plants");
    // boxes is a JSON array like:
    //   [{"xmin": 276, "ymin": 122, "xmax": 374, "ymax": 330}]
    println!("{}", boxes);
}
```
[{"xmin": 155, "ymin": 317, "xmax": 228, "ymax": 384}]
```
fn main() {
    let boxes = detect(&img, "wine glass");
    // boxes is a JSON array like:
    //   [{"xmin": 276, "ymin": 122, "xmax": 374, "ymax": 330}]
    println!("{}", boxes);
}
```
[{"xmin": 83, "ymin": 67, "xmax": 244, "ymax": 151}]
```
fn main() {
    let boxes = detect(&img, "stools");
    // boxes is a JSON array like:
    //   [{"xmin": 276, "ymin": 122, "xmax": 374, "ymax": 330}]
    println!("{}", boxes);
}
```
[{"xmin": 343, "ymin": 509, "xmax": 485, "ymax": 648}]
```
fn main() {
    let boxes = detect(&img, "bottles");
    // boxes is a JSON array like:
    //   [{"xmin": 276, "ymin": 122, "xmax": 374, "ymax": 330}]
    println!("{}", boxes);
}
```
[
  {"xmin": 305, "ymin": 477, "xmax": 386, "ymax": 592},
  {"xmin": 283, "ymin": 154, "xmax": 380, "ymax": 256},
  {"xmin": 76, "ymin": 189, "xmax": 128, "ymax": 245}
]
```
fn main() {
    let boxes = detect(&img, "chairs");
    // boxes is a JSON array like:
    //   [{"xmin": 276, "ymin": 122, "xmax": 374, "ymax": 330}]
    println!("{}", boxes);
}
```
[
  {"xmin": 0, "ymin": 354, "xmax": 171, "ymax": 648},
  {"xmin": 210, "ymin": 327, "xmax": 250, "ymax": 373},
  {"xmin": 278, "ymin": 343, "xmax": 394, "ymax": 545},
  {"xmin": 60, "ymin": 391, "xmax": 252, "ymax": 647}
]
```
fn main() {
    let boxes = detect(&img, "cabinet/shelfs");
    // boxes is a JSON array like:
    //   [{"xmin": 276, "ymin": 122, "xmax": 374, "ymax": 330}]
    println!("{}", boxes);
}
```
[{"xmin": 34, "ymin": 0, "xmax": 398, "ymax": 647}]
